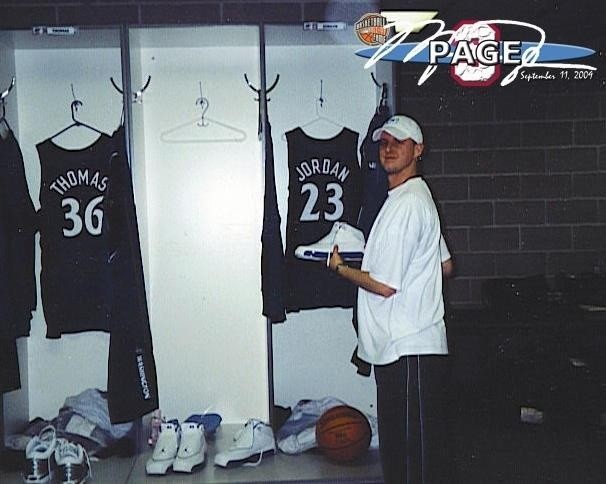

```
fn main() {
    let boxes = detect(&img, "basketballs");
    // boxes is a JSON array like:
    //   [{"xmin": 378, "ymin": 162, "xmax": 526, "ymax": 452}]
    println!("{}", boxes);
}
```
[{"xmin": 316, "ymin": 406, "xmax": 371, "ymax": 460}]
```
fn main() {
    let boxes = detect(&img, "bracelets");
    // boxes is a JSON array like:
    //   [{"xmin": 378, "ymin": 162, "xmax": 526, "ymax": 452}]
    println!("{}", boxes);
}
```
[{"xmin": 335, "ymin": 263, "xmax": 344, "ymax": 274}]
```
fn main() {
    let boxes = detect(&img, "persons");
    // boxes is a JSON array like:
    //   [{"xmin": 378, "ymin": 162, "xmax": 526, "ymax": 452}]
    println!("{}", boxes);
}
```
[{"xmin": 320, "ymin": 113, "xmax": 454, "ymax": 482}]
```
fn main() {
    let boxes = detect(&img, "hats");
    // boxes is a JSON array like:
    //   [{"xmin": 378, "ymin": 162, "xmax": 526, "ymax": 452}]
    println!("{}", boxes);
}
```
[{"xmin": 372, "ymin": 114, "xmax": 424, "ymax": 144}]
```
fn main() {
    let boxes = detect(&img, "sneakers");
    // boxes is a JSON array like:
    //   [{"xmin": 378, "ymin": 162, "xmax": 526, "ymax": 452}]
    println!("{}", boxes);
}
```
[
  {"xmin": 172, "ymin": 419, "xmax": 207, "ymax": 473},
  {"xmin": 19, "ymin": 425, "xmax": 93, "ymax": 484},
  {"xmin": 145, "ymin": 418, "xmax": 181, "ymax": 476},
  {"xmin": 294, "ymin": 222, "xmax": 366, "ymax": 264},
  {"xmin": 214, "ymin": 418, "xmax": 277, "ymax": 468}
]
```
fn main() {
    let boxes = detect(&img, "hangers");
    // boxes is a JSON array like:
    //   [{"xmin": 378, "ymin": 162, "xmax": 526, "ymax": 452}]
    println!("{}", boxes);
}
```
[
  {"xmin": 50, "ymin": 100, "xmax": 101, "ymax": 139},
  {"xmin": 376, "ymin": 83, "xmax": 386, "ymax": 114},
  {"xmin": 0, "ymin": 92, "xmax": 10, "ymax": 133},
  {"xmin": 160, "ymin": 97, "xmax": 247, "ymax": 142},
  {"xmin": 281, "ymin": 97, "xmax": 343, "ymax": 141}
]
